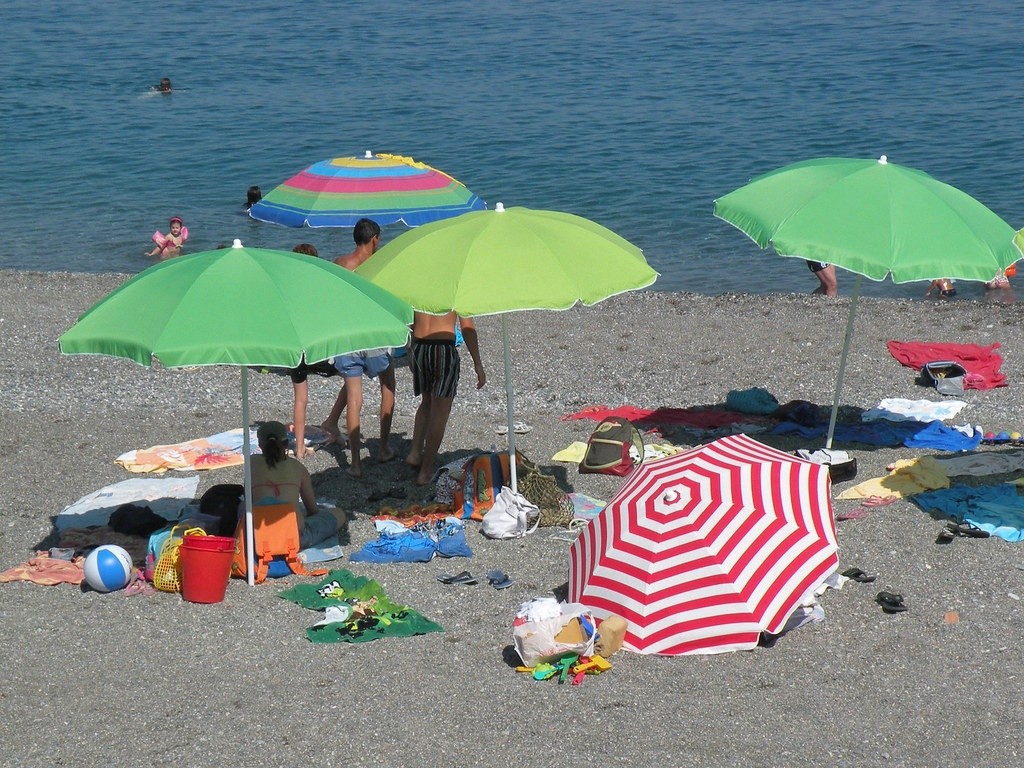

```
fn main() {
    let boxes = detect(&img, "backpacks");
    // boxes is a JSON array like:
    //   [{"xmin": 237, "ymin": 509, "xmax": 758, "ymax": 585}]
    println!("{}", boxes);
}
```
[{"xmin": 580, "ymin": 416, "xmax": 644, "ymax": 476}]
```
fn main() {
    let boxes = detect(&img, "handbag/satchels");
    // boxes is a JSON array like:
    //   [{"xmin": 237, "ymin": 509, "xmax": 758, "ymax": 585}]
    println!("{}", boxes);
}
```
[
  {"xmin": 727, "ymin": 386, "xmax": 778, "ymax": 413},
  {"xmin": 514, "ymin": 608, "xmax": 595, "ymax": 668},
  {"xmin": 429, "ymin": 448, "xmax": 576, "ymax": 540},
  {"xmin": 920, "ymin": 360, "xmax": 969, "ymax": 396}
]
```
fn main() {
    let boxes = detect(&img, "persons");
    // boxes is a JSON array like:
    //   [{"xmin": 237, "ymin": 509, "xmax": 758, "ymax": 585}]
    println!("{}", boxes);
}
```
[
  {"xmin": 330, "ymin": 218, "xmax": 397, "ymax": 476},
  {"xmin": 242, "ymin": 186, "xmax": 263, "ymax": 208},
  {"xmin": 407, "ymin": 308, "xmax": 486, "ymax": 485},
  {"xmin": 806, "ymin": 259, "xmax": 837, "ymax": 298},
  {"xmin": 243, "ymin": 420, "xmax": 347, "ymax": 549},
  {"xmin": 159, "ymin": 78, "xmax": 173, "ymax": 91},
  {"xmin": 926, "ymin": 279, "xmax": 956, "ymax": 298},
  {"xmin": 144, "ymin": 216, "xmax": 189, "ymax": 260},
  {"xmin": 985, "ymin": 275, "xmax": 1011, "ymax": 289},
  {"xmin": 292, "ymin": 243, "xmax": 347, "ymax": 459}
]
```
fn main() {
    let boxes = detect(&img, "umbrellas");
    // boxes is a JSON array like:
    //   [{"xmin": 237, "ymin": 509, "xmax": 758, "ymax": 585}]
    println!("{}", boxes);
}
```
[
  {"xmin": 350, "ymin": 201, "xmax": 661, "ymax": 493},
  {"xmin": 246, "ymin": 149, "xmax": 487, "ymax": 228},
  {"xmin": 568, "ymin": 434, "xmax": 840, "ymax": 657},
  {"xmin": 57, "ymin": 238, "xmax": 414, "ymax": 588},
  {"xmin": 1012, "ymin": 226, "xmax": 1024, "ymax": 257},
  {"xmin": 712, "ymin": 155, "xmax": 1022, "ymax": 448}
]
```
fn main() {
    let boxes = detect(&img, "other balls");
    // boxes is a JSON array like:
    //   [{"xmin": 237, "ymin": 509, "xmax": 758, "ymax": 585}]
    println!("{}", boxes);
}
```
[{"xmin": 83, "ymin": 544, "xmax": 134, "ymax": 593}]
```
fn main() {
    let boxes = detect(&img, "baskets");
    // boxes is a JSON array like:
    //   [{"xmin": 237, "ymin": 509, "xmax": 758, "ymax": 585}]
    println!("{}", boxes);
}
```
[{"xmin": 153, "ymin": 524, "xmax": 207, "ymax": 593}]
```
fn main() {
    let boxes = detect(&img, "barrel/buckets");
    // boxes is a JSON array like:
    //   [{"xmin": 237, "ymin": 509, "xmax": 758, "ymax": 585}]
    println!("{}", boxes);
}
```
[{"xmin": 177, "ymin": 535, "xmax": 236, "ymax": 604}]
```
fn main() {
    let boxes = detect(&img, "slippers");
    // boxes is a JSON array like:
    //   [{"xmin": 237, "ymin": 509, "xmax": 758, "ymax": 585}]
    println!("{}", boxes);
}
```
[
  {"xmin": 939, "ymin": 526, "xmax": 960, "ymax": 540},
  {"xmin": 493, "ymin": 420, "xmax": 533, "ymax": 434},
  {"xmin": 483, "ymin": 570, "xmax": 514, "ymax": 588},
  {"xmin": 946, "ymin": 522, "xmax": 990, "ymax": 538},
  {"xmin": 369, "ymin": 489, "xmax": 387, "ymax": 502},
  {"xmin": 389, "ymin": 486, "xmax": 406, "ymax": 498},
  {"xmin": 841, "ymin": 568, "xmax": 875, "ymax": 583},
  {"xmin": 877, "ymin": 591, "xmax": 908, "ymax": 612},
  {"xmin": 436, "ymin": 570, "xmax": 477, "ymax": 584}
]
